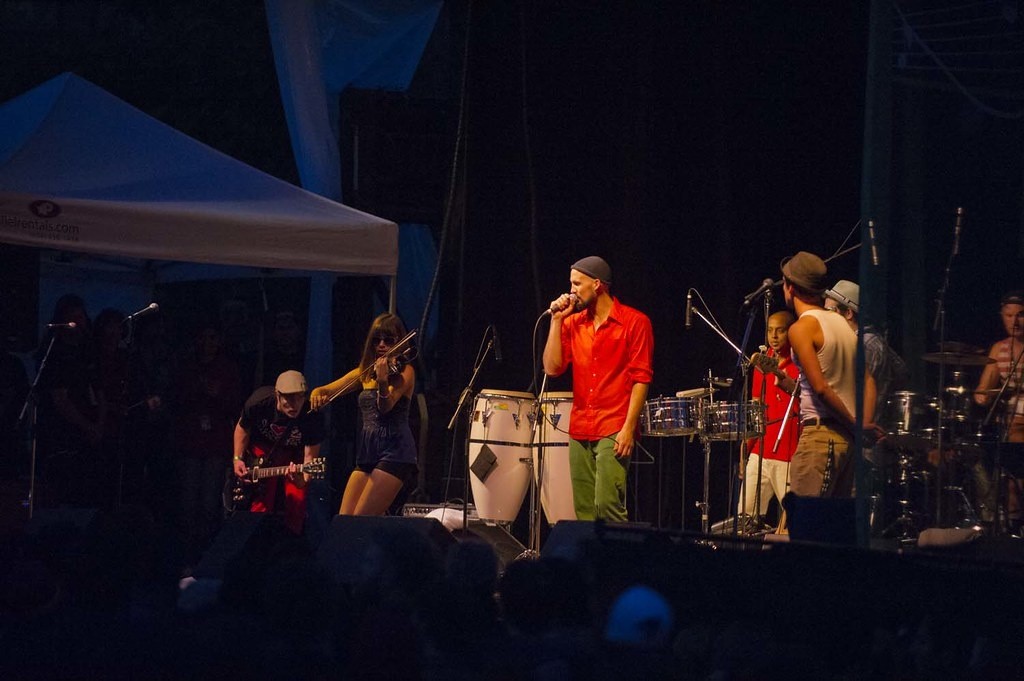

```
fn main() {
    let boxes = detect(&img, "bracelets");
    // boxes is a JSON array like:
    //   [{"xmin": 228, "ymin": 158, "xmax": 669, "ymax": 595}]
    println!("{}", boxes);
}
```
[{"xmin": 377, "ymin": 390, "xmax": 390, "ymax": 399}]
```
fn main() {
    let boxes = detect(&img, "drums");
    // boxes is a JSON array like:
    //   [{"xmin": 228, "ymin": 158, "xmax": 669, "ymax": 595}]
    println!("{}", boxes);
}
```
[
  {"xmin": 640, "ymin": 397, "xmax": 710, "ymax": 436},
  {"xmin": 698, "ymin": 400, "xmax": 768, "ymax": 445},
  {"xmin": 878, "ymin": 389, "xmax": 948, "ymax": 450},
  {"xmin": 467, "ymin": 388, "xmax": 539, "ymax": 525},
  {"xmin": 870, "ymin": 471, "xmax": 947, "ymax": 539},
  {"xmin": 947, "ymin": 414, "xmax": 1014, "ymax": 444},
  {"xmin": 531, "ymin": 390, "xmax": 579, "ymax": 529}
]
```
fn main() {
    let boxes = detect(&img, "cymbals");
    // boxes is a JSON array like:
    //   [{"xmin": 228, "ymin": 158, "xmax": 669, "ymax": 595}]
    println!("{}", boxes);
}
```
[
  {"xmin": 920, "ymin": 353, "xmax": 997, "ymax": 365},
  {"xmin": 933, "ymin": 338, "xmax": 987, "ymax": 354}
]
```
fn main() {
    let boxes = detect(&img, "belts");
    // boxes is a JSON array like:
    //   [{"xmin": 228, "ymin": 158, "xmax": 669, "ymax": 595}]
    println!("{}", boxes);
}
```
[{"xmin": 803, "ymin": 418, "xmax": 825, "ymax": 425}]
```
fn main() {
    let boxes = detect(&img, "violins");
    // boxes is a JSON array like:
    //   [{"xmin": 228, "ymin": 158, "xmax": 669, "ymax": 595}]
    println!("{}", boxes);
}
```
[{"xmin": 367, "ymin": 349, "xmax": 410, "ymax": 387}]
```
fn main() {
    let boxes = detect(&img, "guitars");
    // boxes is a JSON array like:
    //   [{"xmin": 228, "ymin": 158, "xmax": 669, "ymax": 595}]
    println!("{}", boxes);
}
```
[
  {"xmin": 748, "ymin": 344, "xmax": 800, "ymax": 395},
  {"xmin": 221, "ymin": 454, "xmax": 325, "ymax": 513}
]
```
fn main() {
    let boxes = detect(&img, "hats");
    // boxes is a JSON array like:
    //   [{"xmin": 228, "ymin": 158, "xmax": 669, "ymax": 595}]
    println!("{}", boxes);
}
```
[
  {"xmin": 999, "ymin": 290, "xmax": 1024, "ymax": 306},
  {"xmin": 274, "ymin": 370, "xmax": 308, "ymax": 394},
  {"xmin": 570, "ymin": 256, "xmax": 613, "ymax": 286},
  {"xmin": 780, "ymin": 251, "xmax": 829, "ymax": 293},
  {"xmin": 825, "ymin": 279, "xmax": 859, "ymax": 313}
]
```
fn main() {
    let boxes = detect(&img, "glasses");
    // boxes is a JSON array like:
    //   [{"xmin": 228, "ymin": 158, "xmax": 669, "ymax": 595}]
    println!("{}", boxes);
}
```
[{"xmin": 371, "ymin": 337, "xmax": 394, "ymax": 346}]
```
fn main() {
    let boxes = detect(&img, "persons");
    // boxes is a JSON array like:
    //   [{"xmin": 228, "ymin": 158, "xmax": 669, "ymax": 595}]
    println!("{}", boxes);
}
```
[
  {"xmin": 426, "ymin": 536, "xmax": 670, "ymax": 681},
  {"xmin": 542, "ymin": 256, "xmax": 655, "ymax": 521},
  {"xmin": 738, "ymin": 252, "xmax": 910, "ymax": 536},
  {"xmin": 310, "ymin": 312, "xmax": 419, "ymax": 516},
  {"xmin": 973, "ymin": 291, "xmax": 1024, "ymax": 522},
  {"xmin": 43, "ymin": 307, "xmax": 306, "ymax": 541}
]
